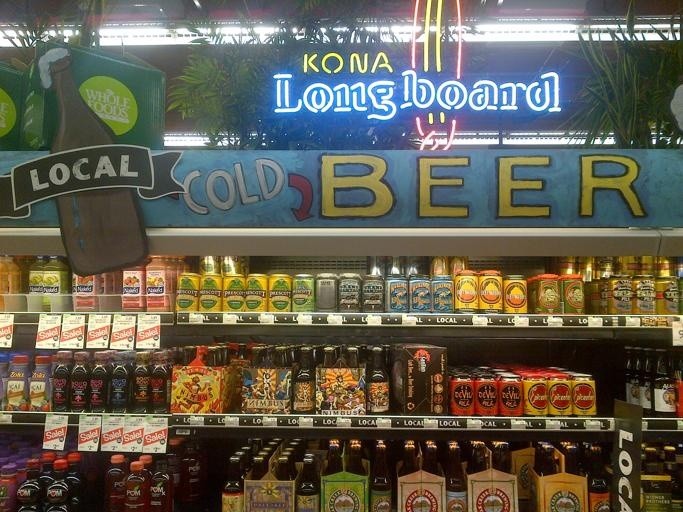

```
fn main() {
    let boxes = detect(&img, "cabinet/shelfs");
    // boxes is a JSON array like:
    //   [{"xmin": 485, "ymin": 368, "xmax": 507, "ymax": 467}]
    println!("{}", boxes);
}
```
[{"xmin": 0, "ymin": 227, "xmax": 683, "ymax": 511}]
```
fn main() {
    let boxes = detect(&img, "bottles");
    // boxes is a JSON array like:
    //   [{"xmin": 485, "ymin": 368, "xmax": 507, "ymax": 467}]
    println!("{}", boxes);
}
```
[
  {"xmin": 367, "ymin": 347, "xmax": 390, "ymax": 415},
  {"xmin": 504, "ymin": 275, "xmax": 529, "ymax": 313},
  {"xmin": 121, "ymin": 266, "xmax": 146, "ymax": 310},
  {"xmin": 560, "ymin": 439, "xmax": 580, "ymax": 475},
  {"xmin": 176, "ymin": 272, "xmax": 199, "ymax": 312},
  {"xmin": 316, "ymin": 343, "xmax": 367, "ymax": 367},
  {"xmin": 475, "ymin": 374, "xmax": 499, "ymax": 415},
  {"xmin": 110, "ymin": 351, "xmax": 131, "ymax": 413},
  {"xmin": 524, "ymin": 375, "xmax": 548, "ymax": 416},
  {"xmin": 346, "ymin": 440, "xmax": 365, "ymax": 476},
  {"xmin": 559, "ymin": 256, "xmax": 577, "ymax": 276},
  {"xmin": 71, "ymin": 352, "xmax": 90, "ymax": 412},
  {"xmin": 561, "ymin": 274, "xmax": 585, "ymax": 314},
  {"xmin": 292, "ymin": 347, "xmax": 316, "ymax": 415},
  {"xmin": 200, "ymin": 273, "xmax": 222, "ymax": 312},
  {"xmin": 381, "ymin": 344, "xmax": 391, "ymax": 374},
  {"xmin": 222, "ymin": 256, "xmax": 242, "ymax": 274},
  {"xmin": 178, "ymin": 256, "xmax": 190, "ymax": 272},
  {"xmin": 172, "ymin": 256, "xmax": 182, "ymax": 273},
  {"xmin": 296, "ymin": 458, "xmax": 319, "ymax": 512},
  {"xmin": 32, "ymin": 356, "xmax": 53, "ymax": 412},
  {"xmin": 164, "ymin": 256, "xmax": 176, "ymax": 312},
  {"xmin": 404, "ymin": 256, "xmax": 422, "ymax": 277},
  {"xmin": 616, "ymin": 256, "xmax": 638, "ymax": 278},
  {"xmin": 72, "ymin": 272, "xmax": 121, "ymax": 311},
  {"xmin": 638, "ymin": 349, "xmax": 653, "ymax": 415},
  {"xmin": 499, "ymin": 375, "xmax": 524, "ymax": 416},
  {"xmin": 89, "ymin": 352, "xmax": 110, "ymax": 412},
  {"xmin": 131, "ymin": 352, "xmax": 152, "ymax": 413},
  {"xmin": 586, "ymin": 280, "xmax": 609, "ymax": 314},
  {"xmin": 54, "ymin": 351, "xmax": 71, "ymax": 412},
  {"xmin": 43, "ymin": 254, "xmax": 69, "ymax": 311},
  {"xmin": 8, "ymin": 356, "xmax": 31, "ymax": 412},
  {"xmin": 658, "ymin": 257, "xmax": 675, "ymax": 278},
  {"xmin": 629, "ymin": 346, "xmax": 643, "ymax": 406},
  {"xmin": 17, "ymin": 460, "xmax": 45, "ymax": 512},
  {"xmin": 663, "ymin": 446, "xmax": 681, "ymax": 499},
  {"xmin": 455, "ymin": 270, "xmax": 479, "ymax": 314},
  {"xmin": 429, "ymin": 255, "xmax": 450, "ymax": 277},
  {"xmin": 363, "ymin": 275, "xmax": 385, "ymax": 313},
  {"xmin": 398, "ymin": 444, "xmax": 419, "ymax": 475},
  {"xmin": 370, "ymin": 445, "xmax": 392, "ymax": 512},
  {"xmin": 385, "ymin": 255, "xmax": 403, "ymax": 276},
  {"xmin": 607, "ymin": 278, "xmax": 634, "ymax": 315},
  {"xmin": 199, "ymin": 256, "xmax": 221, "ymax": 273},
  {"xmin": 292, "ymin": 274, "xmax": 315, "ymax": 312},
  {"xmin": 634, "ymin": 275, "xmax": 657, "ymax": 315},
  {"xmin": 538, "ymin": 445, "xmax": 557, "ymax": 476},
  {"xmin": 236, "ymin": 437, "xmax": 302, "ymax": 482},
  {"xmin": 0, "ymin": 463, "xmax": 18, "ymax": 511},
  {"xmin": 168, "ymin": 439, "xmax": 181, "ymax": 495},
  {"xmin": 28, "ymin": 254, "xmax": 48, "ymax": 311},
  {"xmin": 65, "ymin": 453, "xmax": 83, "ymax": 512},
  {"xmin": 104, "ymin": 454, "xmax": 128, "ymax": 512},
  {"xmin": 315, "ymin": 274, "xmax": 338, "ymax": 312},
  {"xmin": 639, "ymin": 256, "xmax": 658, "ymax": 277},
  {"xmin": 480, "ymin": 269, "xmax": 503, "ymax": 314},
  {"xmin": 494, "ymin": 443, "xmax": 511, "ymax": 473},
  {"xmin": 41, "ymin": 452, "xmax": 56, "ymax": 481},
  {"xmin": 423, "ymin": 445, "xmax": 441, "ymax": 476},
  {"xmin": 572, "ymin": 375, "xmax": 597, "ymax": 416},
  {"xmin": 222, "ymin": 274, "xmax": 246, "ymax": 312},
  {"xmin": 469, "ymin": 442, "xmax": 488, "ymax": 473},
  {"xmin": 125, "ymin": 461, "xmax": 149, "ymax": 512},
  {"xmin": 433, "ymin": 275, "xmax": 455, "ymax": 313},
  {"xmin": 152, "ymin": 352, "xmax": 168, "ymax": 413},
  {"xmin": 246, "ymin": 273, "xmax": 268, "ymax": 312},
  {"xmin": 366, "ymin": 255, "xmax": 385, "ymax": 275},
  {"xmin": 268, "ymin": 273, "xmax": 292, "ymax": 312},
  {"xmin": 41, "ymin": 460, "xmax": 72, "ymax": 512},
  {"xmin": 177, "ymin": 343, "xmax": 291, "ymax": 367},
  {"xmin": 410, "ymin": 274, "xmax": 433, "ymax": 313},
  {"xmin": 589, "ymin": 447, "xmax": 610, "ymax": 512},
  {"xmin": 527, "ymin": 273, "xmax": 560, "ymax": 314},
  {"xmin": 386, "ymin": 274, "xmax": 409, "ymax": 314},
  {"xmin": 596, "ymin": 256, "xmax": 616, "ymax": 279},
  {"xmin": 450, "ymin": 256, "xmax": 469, "ymax": 276},
  {"xmin": 446, "ymin": 446, "xmax": 466, "ymax": 512},
  {"xmin": 146, "ymin": 255, "xmax": 169, "ymax": 312},
  {"xmin": 548, "ymin": 373, "xmax": 573, "ymax": 415},
  {"xmin": 448, "ymin": 374, "xmax": 475, "ymax": 415},
  {"xmin": 623, "ymin": 346, "xmax": 633, "ymax": 402},
  {"xmin": 657, "ymin": 276, "xmax": 679, "ymax": 315},
  {"xmin": 150, "ymin": 453, "xmax": 174, "ymax": 512},
  {"xmin": 222, "ymin": 456, "xmax": 244, "ymax": 512},
  {"xmin": 645, "ymin": 448, "xmax": 662, "ymax": 493},
  {"xmin": 338, "ymin": 273, "xmax": 362, "ymax": 313},
  {"xmin": 324, "ymin": 439, "xmax": 342, "ymax": 476},
  {"xmin": 182, "ymin": 439, "xmax": 202, "ymax": 501},
  {"xmin": 577, "ymin": 256, "xmax": 596, "ymax": 280},
  {"xmin": 651, "ymin": 349, "xmax": 676, "ymax": 415}
]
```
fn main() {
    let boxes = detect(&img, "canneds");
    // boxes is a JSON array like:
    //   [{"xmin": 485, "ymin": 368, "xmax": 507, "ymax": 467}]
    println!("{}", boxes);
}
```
[{"xmin": 0, "ymin": 255, "xmax": 22, "ymax": 312}]
